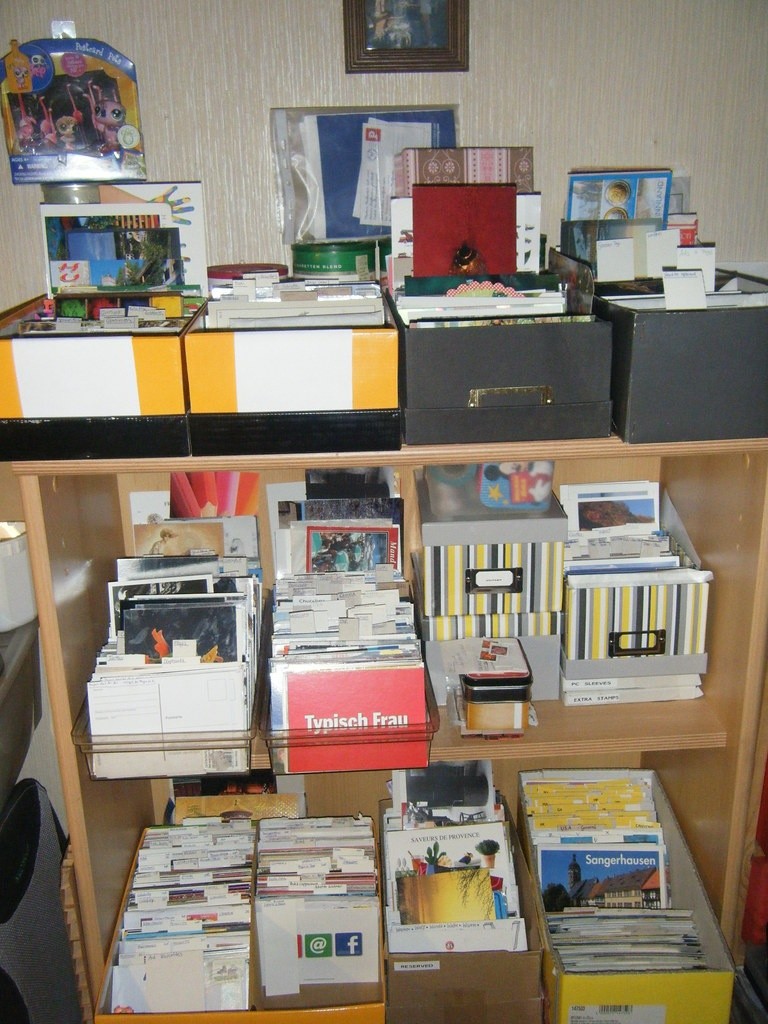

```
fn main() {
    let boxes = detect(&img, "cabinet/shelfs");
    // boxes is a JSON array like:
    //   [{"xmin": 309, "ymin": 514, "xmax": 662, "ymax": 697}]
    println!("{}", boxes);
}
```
[{"xmin": 19, "ymin": 435, "xmax": 768, "ymax": 1024}]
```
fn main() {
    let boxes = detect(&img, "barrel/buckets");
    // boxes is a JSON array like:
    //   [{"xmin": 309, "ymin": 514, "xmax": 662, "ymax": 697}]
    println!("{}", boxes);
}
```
[
  {"xmin": 0, "ymin": 521, "xmax": 38, "ymax": 632},
  {"xmin": 290, "ymin": 239, "xmax": 391, "ymax": 276},
  {"xmin": 207, "ymin": 263, "xmax": 289, "ymax": 294}
]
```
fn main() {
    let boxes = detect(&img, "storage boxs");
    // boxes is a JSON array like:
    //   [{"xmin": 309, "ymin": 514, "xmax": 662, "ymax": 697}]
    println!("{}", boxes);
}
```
[
  {"xmin": 565, "ymin": 531, "xmax": 712, "ymax": 672},
  {"xmin": 517, "ymin": 767, "xmax": 736, "ymax": 1024},
  {"xmin": 95, "ymin": 816, "xmax": 386, "ymax": 1023},
  {"xmin": 378, "ymin": 792, "xmax": 544, "ymax": 1024},
  {"xmin": 0, "ymin": 295, "xmax": 210, "ymax": 419},
  {"xmin": 460, "ymin": 671, "xmax": 533, "ymax": 732},
  {"xmin": 70, "ymin": 590, "xmax": 262, "ymax": 780},
  {"xmin": 409, "ymin": 583, "xmax": 562, "ymax": 707},
  {"xmin": 593, "ymin": 268, "xmax": 768, "ymax": 445},
  {"xmin": 416, "ymin": 470, "xmax": 568, "ymax": 615},
  {"xmin": 389, "ymin": 290, "xmax": 612, "ymax": 442},
  {"xmin": 263, "ymin": 592, "xmax": 440, "ymax": 771},
  {"xmin": 185, "ymin": 294, "xmax": 399, "ymax": 411}
]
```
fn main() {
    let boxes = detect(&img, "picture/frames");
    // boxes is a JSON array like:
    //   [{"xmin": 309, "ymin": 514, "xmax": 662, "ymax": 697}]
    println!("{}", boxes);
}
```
[{"xmin": 344, "ymin": 0, "xmax": 469, "ymax": 75}]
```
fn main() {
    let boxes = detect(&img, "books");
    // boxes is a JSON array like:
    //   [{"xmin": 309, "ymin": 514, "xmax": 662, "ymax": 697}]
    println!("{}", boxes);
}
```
[{"xmin": 27, "ymin": 139, "xmax": 757, "ymax": 1010}]
